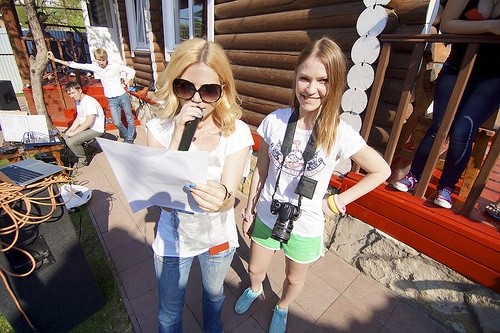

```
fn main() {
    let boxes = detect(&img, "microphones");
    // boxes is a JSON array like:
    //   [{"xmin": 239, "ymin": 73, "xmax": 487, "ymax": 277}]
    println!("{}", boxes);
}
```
[{"xmin": 178, "ymin": 105, "xmax": 205, "ymax": 150}]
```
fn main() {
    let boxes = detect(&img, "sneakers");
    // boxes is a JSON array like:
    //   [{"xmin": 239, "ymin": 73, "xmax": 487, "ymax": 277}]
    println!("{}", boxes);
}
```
[
  {"xmin": 393, "ymin": 172, "xmax": 417, "ymax": 192},
  {"xmin": 434, "ymin": 186, "xmax": 452, "ymax": 209},
  {"xmin": 235, "ymin": 284, "xmax": 263, "ymax": 313},
  {"xmin": 269, "ymin": 301, "xmax": 288, "ymax": 333}
]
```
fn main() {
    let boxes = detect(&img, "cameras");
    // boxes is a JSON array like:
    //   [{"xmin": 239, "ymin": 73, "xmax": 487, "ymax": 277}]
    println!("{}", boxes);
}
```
[{"xmin": 271, "ymin": 199, "xmax": 300, "ymax": 243}]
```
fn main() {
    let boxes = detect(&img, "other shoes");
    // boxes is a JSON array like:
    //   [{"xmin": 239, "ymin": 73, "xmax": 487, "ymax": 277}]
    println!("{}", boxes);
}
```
[
  {"xmin": 73, "ymin": 161, "xmax": 88, "ymax": 169},
  {"xmin": 122, "ymin": 137, "xmax": 134, "ymax": 144}
]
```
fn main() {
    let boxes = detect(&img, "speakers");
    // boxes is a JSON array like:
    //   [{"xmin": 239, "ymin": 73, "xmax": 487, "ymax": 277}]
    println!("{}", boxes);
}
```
[{"xmin": 0, "ymin": 187, "xmax": 107, "ymax": 333}]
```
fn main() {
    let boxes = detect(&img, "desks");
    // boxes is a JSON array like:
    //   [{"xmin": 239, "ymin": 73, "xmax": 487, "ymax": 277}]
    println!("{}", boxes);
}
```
[{"xmin": 0, "ymin": 145, "xmax": 69, "ymax": 175}]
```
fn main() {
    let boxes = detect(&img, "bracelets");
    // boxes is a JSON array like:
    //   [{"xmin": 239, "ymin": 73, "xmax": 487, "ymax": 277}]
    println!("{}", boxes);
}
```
[
  {"xmin": 221, "ymin": 184, "xmax": 231, "ymax": 208},
  {"xmin": 241, "ymin": 208, "xmax": 256, "ymax": 221},
  {"xmin": 327, "ymin": 194, "xmax": 346, "ymax": 214}
]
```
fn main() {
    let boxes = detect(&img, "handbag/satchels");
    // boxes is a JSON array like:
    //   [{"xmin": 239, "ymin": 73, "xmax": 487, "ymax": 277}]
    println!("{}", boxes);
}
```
[{"xmin": 58, "ymin": 184, "xmax": 92, "ymax": 210}]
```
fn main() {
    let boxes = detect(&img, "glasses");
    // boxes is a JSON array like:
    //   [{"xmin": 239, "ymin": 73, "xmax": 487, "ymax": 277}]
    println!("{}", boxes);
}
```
[{"xmin": 173, "ymin": 78, "xmax": 225, "ymax": 103}]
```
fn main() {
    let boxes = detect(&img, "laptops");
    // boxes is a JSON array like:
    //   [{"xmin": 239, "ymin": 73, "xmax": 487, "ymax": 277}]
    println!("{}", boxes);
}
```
[{"xmin": 0, "ymin": 158, "xmax": 65, "ymax": 187}]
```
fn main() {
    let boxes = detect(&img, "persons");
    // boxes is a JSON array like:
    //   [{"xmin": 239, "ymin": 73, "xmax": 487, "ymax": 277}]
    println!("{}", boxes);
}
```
[
  {"xmin": 394, "ymin": 0, "xmax": 500, "ymax": 219},
  {"xmin": 47, "ymin": 49, "xmax": 136, "ymax": 144},
  {"xmin": 63, "ymin": 82, "xmax": 104, "ymax": 167},
  {"xmin": 64, "ymin": 31, "xmax": 91, "ymax": 76},
  {"xmin": 235, "ymin": 38, "xmax": 391, "ymax": 333},
  {"xmin": 144, "ymin": 38, "xmax": 254, "ymax": 333}
]
